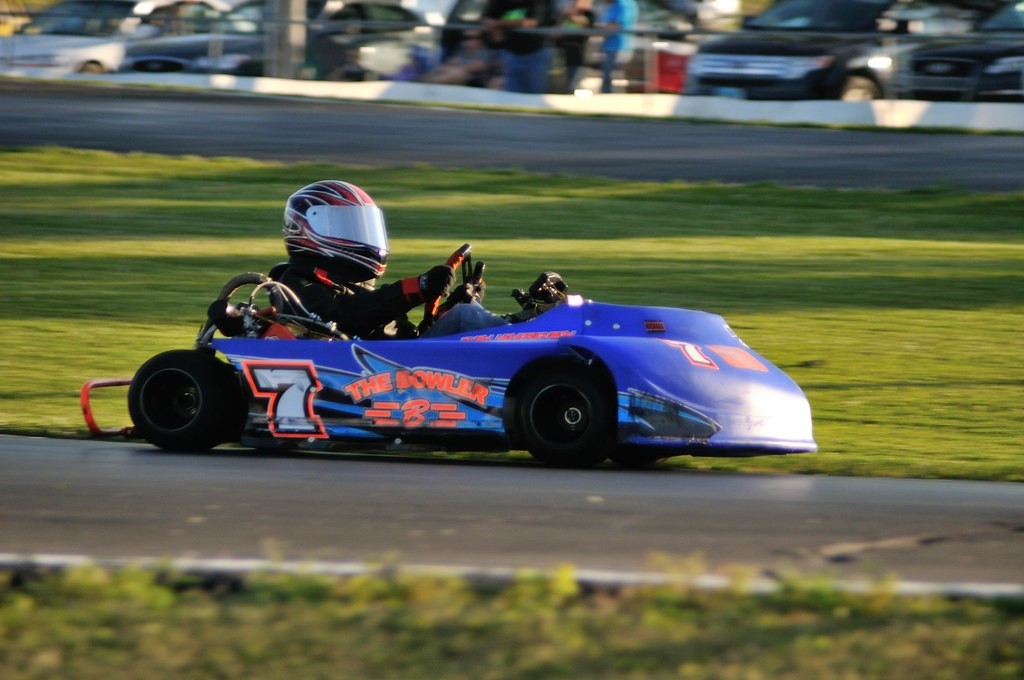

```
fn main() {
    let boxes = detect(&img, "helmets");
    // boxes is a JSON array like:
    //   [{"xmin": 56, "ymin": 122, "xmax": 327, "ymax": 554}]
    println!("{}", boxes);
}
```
[{"xmin": 282, "ymin": 181, "xmax": 390, "ymax": 277}]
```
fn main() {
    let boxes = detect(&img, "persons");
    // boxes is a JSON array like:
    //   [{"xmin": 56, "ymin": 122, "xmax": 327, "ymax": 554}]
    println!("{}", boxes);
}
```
[
  {"xmin": 276, "ymin": 180, "xmax": 512, "ymax": 341},
  {"xmin": 423, "ymin": 0, "xmax": 638, "ymax": 94}
]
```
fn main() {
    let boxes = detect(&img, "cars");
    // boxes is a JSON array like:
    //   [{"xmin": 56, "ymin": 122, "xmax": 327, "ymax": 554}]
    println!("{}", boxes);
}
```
[
  {"xmin": 0, "ymin": 2, "xmax": 165, "ymax": 76},
  {"xmin": 892, "ymin": 0, "xmax": 1024, "ymax": 97},
  {"xmin": 686, "ymin": 0, "xmax": 917, "ymax": 102},
  {"xmin": 122, "ymin": 2, "xmax": 451, "ymax": 74}
]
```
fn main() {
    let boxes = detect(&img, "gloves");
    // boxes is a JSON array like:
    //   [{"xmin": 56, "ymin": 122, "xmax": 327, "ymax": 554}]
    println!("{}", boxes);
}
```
[
  {"xmin": 447, "ymin": 281, "xmax": 486, "ymax": 307},
  {"xmin": 419, "ymin": 265, "xmax": 456, "ymax": 303}
]
